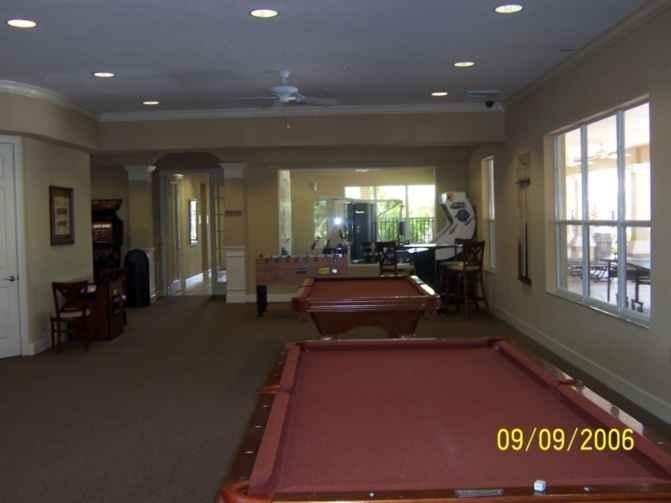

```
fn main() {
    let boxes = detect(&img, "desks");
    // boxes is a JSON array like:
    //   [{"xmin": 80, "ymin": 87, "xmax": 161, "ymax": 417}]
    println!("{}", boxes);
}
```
[
  {"xmin": 375, "ymin": 241, "xmax": 398, "ymax": 276},
  {"xmin": 292, "ymin": 271, "xmax": 441, "ymax": 337},
  {"xmin": 253, "ymin": 254, "xmax": 346, "ymax": 317},
  {"xmin": 214, "ymin": 335, "xmax": 671, "ymax": 503}
]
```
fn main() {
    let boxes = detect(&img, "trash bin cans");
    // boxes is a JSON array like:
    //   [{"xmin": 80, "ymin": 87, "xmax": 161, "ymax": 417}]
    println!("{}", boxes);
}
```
[{"xmin": 124, "ymin": 249, "xmax": 150, "ymax": 307}]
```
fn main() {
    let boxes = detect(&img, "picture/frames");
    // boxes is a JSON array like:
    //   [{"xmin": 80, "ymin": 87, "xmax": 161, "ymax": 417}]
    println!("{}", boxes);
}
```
[{"xmin": 49, "ymin": 186, "xmax": 75, "ymax": 245}]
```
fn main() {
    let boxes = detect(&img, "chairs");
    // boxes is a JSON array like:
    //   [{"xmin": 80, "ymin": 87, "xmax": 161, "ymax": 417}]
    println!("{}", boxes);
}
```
[
  {"xmin": 50, "ymin": 281, "xmax": 89, "ymax": 352},
  {"xmin": 441, "ymin": 239, "xmax": 489, "ymax": 322},
  {"xmin": 435, "ymin": 239, "xmax": 471, "ymax": 298}
]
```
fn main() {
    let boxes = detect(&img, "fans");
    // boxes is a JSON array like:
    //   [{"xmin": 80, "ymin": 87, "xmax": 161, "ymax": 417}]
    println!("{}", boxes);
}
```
[{"xmin": 232, "ymin": 70, "xmax": 336, "ymax": 128}]
